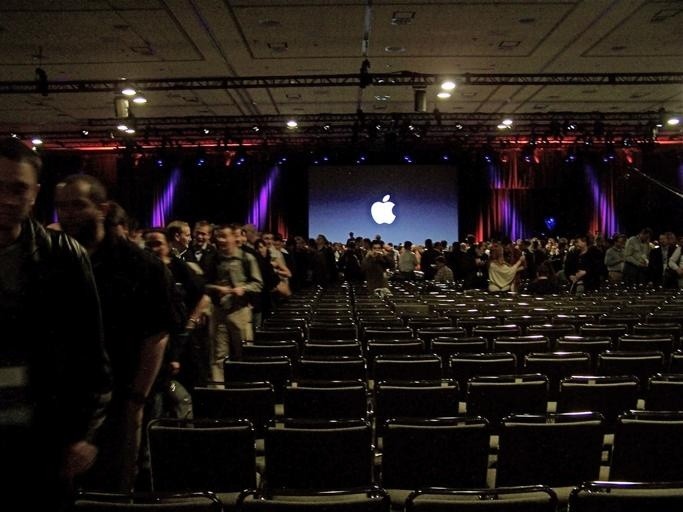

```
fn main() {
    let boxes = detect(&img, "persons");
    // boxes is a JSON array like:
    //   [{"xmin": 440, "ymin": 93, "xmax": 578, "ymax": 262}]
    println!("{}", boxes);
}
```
[
  {"xmin": 291, "ymin": 225, "xmax": 683, "ymax": 298},
  {"xmin": 1, "ymin": 138, "xmax": 291, "ymax": 511}
]
